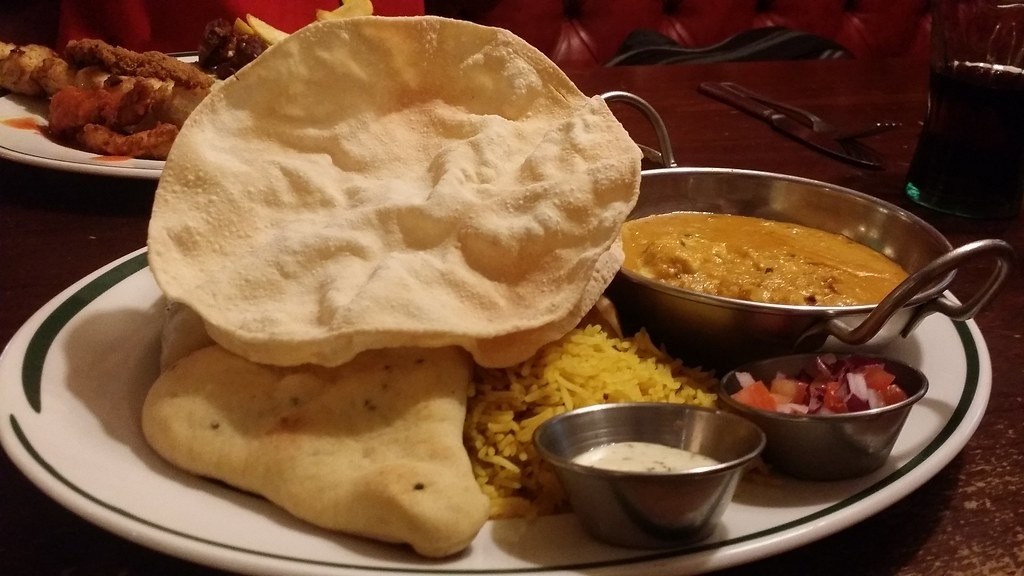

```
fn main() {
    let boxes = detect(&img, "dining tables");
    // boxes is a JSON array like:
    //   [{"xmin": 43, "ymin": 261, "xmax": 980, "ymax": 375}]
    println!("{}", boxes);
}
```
[{"xmin": 0, "ymin": 56, "xmax": 1024, "ymax": 576}]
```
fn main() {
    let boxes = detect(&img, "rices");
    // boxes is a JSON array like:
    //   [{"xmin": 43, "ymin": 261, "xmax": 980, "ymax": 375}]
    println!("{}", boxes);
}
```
[{"xmin": 463, "ymin": 321, "xmax": 745, "ymax": 520}]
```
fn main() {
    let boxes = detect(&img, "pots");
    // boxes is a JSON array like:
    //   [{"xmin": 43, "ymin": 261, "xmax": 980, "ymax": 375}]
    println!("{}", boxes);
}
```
[{"xmin": 603, "ymin": 90, "xmax": 1016, "ymax": 373}]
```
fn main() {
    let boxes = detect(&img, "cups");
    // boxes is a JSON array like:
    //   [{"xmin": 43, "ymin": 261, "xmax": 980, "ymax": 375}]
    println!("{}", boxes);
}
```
[{"xmin": 905, "ymin": 0, "xmax": 1024, "ymax": 221}]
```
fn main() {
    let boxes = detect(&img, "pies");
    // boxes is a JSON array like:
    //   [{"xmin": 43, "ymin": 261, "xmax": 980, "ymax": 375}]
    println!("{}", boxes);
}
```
[{"xmin": 140, "ymin": 16, "xmax": 644, "ymax": 557}]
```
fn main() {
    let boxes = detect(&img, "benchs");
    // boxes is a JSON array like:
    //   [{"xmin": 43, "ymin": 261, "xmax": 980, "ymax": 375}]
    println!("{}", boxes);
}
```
[{"xmin": 422, "ymin": 0, "xmax": 1024, "ymax": 68}]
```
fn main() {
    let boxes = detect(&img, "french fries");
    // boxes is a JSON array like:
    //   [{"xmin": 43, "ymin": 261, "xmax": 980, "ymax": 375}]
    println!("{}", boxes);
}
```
[{"xmin": 234, "ymin": 0, "xmax": 374, "ymax": 49}]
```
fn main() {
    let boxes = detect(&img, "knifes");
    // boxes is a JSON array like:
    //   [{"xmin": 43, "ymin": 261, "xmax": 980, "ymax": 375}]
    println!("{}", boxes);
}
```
[{"xmin": 698, "ymin": 81, "xmax": 886, "ymax": 166}]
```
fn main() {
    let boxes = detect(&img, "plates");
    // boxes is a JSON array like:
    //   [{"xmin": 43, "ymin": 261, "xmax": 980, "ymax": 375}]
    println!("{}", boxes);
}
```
[
  {"xmin": 0, "ymin": 248, "xmax": 992, "ymax": 576},
  {"xmin": 1, "ymin": 51, "xmax": 199, "ymax": 177}
]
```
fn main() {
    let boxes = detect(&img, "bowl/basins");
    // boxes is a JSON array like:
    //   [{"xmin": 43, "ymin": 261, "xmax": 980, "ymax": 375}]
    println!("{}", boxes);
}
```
[
  {"xmin": 533, "ymin": 402, "xmax": 767, "ymax": 549},
  {"xmin": 717, "ymin": 353, "xmax": 929, "ymax": 479}
]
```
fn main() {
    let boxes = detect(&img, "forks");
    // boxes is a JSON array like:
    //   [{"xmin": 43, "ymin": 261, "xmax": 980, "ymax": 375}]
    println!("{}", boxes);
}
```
[{"xmin": 718, "ymin": 82, "xmax": 903, "ymax": 139}]
